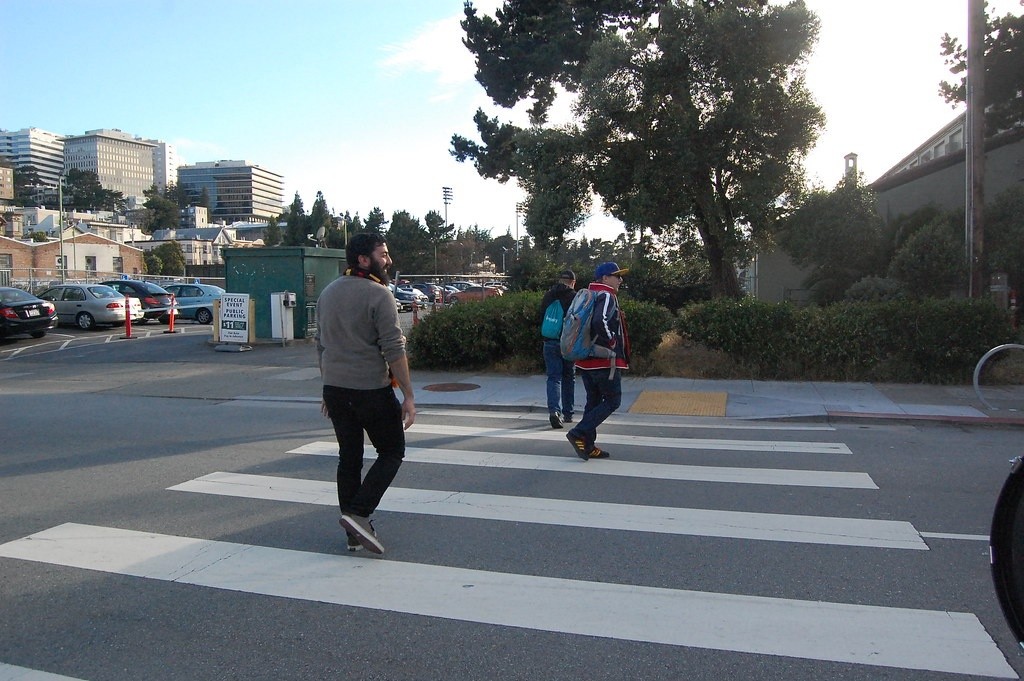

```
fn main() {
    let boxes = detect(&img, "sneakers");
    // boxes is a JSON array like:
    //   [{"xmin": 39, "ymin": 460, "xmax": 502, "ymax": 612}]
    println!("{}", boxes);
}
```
[
  {"xmin": 578, "ymin": 447, "xmax": 609, "ymax": 459},
  {"xmin": 563, "ymin": 412, "xmax": 573, "ymax": 422},
  {"xmin": 549, "ymin": 412, "xmax": 563, "ymax": 428},
  {"xmin": 566, "ymin": 432, "xmax": 589, "ymax": 460},
  {"xmin": 346, "ymin": 533, "xmax": 364, "ymax": 550},
  {"xmin": 342, "ymin": 514, "xmax": 384, "ymax": 554}
]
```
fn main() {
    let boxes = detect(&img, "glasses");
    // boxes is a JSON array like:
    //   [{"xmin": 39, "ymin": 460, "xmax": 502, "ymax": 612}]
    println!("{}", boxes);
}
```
[{"xmin": 606, "ymin": 273, "xmax": 621, "ymax": 280}]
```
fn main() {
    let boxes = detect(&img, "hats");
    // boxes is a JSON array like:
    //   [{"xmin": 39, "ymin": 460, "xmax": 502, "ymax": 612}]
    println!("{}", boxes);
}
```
[
  {"xmin": 560, "ymin": 270, "xmax": 576, "ymax": 280},
  {"xmin": 594, "ymin": 262, "xmax": 629, "ymax": 282}
]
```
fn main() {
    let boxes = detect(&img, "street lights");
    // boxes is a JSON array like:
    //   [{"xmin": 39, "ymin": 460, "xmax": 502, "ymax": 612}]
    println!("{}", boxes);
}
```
[
  {"xmin": 59, "ymin": 175, "xmax": 68, "ymax": 284},
  {"xmin": 515, "ymin": 202, "xmax": 524, "ymax": 263},
  {"xmin": 443, "ymin": 186, "xmax": 453, "ymax": 233}
]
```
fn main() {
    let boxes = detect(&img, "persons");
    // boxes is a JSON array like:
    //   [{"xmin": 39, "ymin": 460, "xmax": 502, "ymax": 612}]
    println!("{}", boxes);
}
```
[
  {"xmin": 196, "ymin": 290, "xmax": 201, "ymax": 296},
  {"xmin": 315, "ymin": 232, "xmax": 417, "ymax": 555},
  {"xmin": 565, "ymin": 262, "xmax": 630, "ymax": 462},
  {"xmin": 540, "ymin": 269, "xmax": 578, "ymax": 428}
]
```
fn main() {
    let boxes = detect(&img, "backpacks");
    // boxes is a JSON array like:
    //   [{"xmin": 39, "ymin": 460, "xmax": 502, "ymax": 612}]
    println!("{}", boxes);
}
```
[
  {"xmin": 543, "ymin": 288, "xmax": 573, "ymax": 338},
  {"xmin": 559, "ymin": 288, "xmax": 617, "ymax": 360}
]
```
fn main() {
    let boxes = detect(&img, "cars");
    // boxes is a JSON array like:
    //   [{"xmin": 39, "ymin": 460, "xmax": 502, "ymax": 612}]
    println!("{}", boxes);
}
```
[
  {"xmin": 158, "ymin": 284, "xmax": 227, "ymax": 324},
  {"xmin": 386, "ymin": 279, "xmax": 510, "ymax": 313},
  {"xmin": 0, "ymin": 287, "xmax": 59, "ymax": 338},
  {"xmin": 36, "ymin": 283, "xmax": 145, "ymax": 331},
  {"xmin": 99, "ymin": 280, "xmax": 179, "ymax": 327}
]
